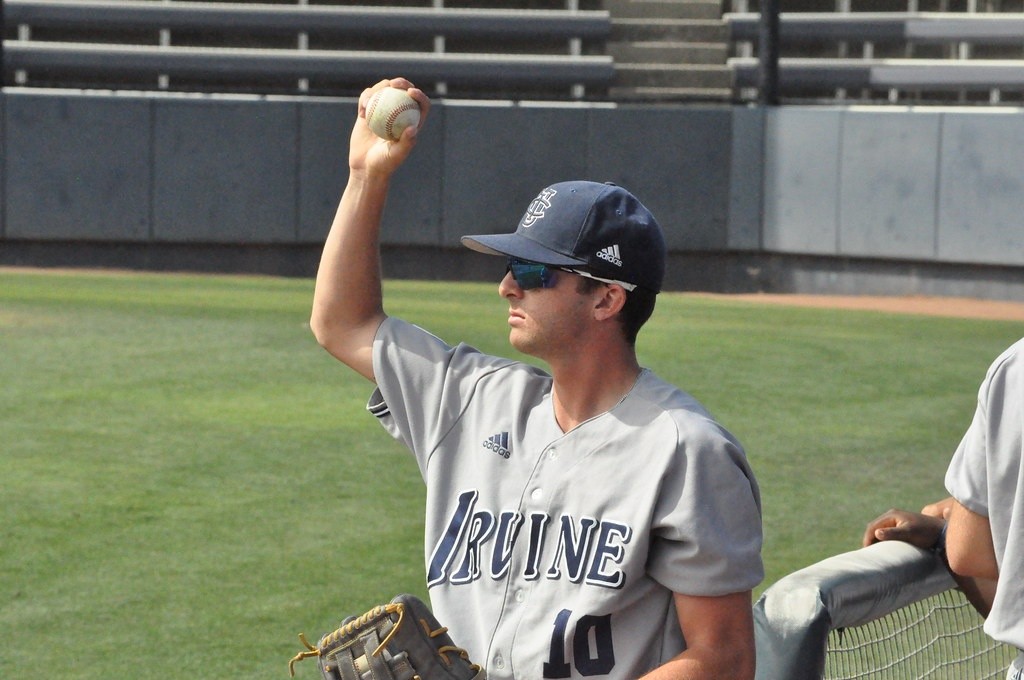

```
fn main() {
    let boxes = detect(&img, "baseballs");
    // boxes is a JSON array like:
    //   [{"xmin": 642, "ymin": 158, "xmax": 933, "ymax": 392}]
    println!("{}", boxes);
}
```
[{"xmin": 365, "ymin": 87, "xmax": 421, "ymax": 140}]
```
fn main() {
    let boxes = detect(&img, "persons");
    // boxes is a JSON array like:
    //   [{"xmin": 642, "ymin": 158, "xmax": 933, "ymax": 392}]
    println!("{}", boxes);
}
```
[
  {"xmin": 864, "ymin": 337, "xmax": 1024, "ymax": 680},
  {"xmin": 308, "ymin": 77, "xmax": 765, "ymax": 680}
]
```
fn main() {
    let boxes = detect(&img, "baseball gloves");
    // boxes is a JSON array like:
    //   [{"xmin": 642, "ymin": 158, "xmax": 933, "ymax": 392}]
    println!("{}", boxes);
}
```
[{"xmin": 288, "ymin": 593, "xmax": 487, "ymax": 680}]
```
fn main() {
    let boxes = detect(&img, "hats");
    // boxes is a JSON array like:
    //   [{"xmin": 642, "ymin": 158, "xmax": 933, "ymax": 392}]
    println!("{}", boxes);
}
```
[{"xmin": 456, "ymin": 180, "xmax": 664, "ymax": 294}]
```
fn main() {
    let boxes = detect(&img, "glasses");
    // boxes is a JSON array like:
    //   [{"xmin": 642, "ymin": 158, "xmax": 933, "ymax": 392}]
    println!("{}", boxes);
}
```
[{"xmin": 502, "ymin": 255, "xmax": 638, "ymax": 292}]
